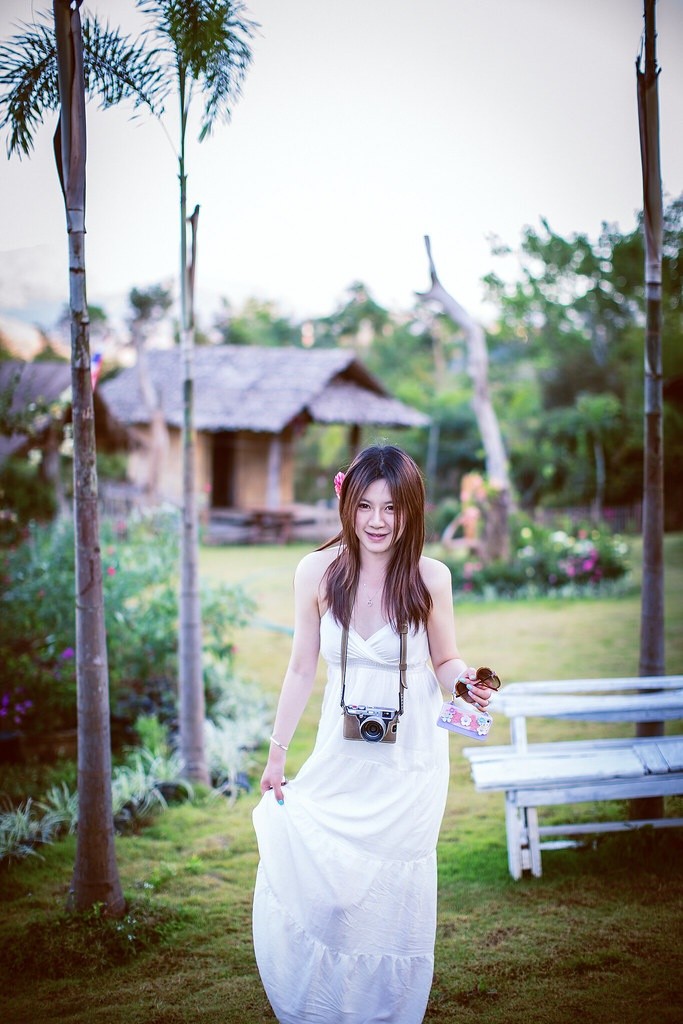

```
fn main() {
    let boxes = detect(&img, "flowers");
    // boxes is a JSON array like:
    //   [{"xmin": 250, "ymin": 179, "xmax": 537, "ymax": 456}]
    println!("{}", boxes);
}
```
[{"xmin": 335, "ymin": 472, "xmax": 345, "ymax": 498}]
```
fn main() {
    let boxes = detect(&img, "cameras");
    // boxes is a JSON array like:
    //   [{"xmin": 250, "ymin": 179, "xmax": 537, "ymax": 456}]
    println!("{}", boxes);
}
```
[{"xmin": 344, "ymin": 706, "xmax": 397, "ymax": 743}]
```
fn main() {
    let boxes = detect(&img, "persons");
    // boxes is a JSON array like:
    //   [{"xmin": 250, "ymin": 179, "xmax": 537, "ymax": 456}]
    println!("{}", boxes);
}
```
[{"xmin": 250, "ymin": 445, "xmax": 491, "ymax": 1024}]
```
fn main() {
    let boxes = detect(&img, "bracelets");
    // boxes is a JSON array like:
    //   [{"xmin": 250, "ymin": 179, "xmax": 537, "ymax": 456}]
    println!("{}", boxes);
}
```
[{"xmin": 269, "ymin": 736, "xmax": 288, "ymax": 750}]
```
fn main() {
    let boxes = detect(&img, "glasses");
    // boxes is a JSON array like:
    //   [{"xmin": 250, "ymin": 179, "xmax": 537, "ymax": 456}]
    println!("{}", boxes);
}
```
[{"xmin": 454, "ymin": 668, "xmax": 501, "ymax": 704}]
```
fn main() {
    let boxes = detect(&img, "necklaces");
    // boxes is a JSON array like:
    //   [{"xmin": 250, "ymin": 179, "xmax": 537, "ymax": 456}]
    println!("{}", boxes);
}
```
[{"xmin": 358, "ymin": 573, "xmax": 383, "ymax": 607}]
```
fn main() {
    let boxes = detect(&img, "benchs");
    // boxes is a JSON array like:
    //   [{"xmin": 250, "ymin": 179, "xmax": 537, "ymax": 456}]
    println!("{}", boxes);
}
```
[{"xmin": 464, "ymin": 675, "xmax": 683, "ymax": 881}]
